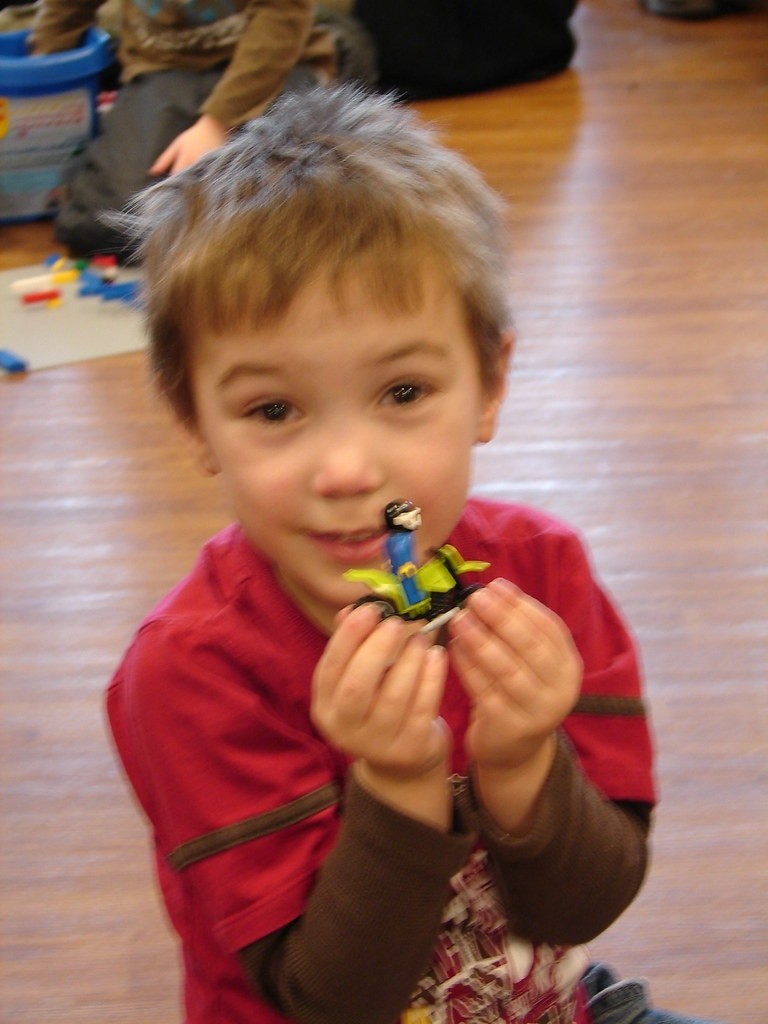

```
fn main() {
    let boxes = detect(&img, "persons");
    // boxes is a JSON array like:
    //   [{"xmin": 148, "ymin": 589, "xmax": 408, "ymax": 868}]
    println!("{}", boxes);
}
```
[
  {"xmin": 102, "ymin": 79, "xmax": 722, "ymax": 1024},
  {"xmin": 25, "ymin": 1, "xmax": 356, "ymax": 270}
]
